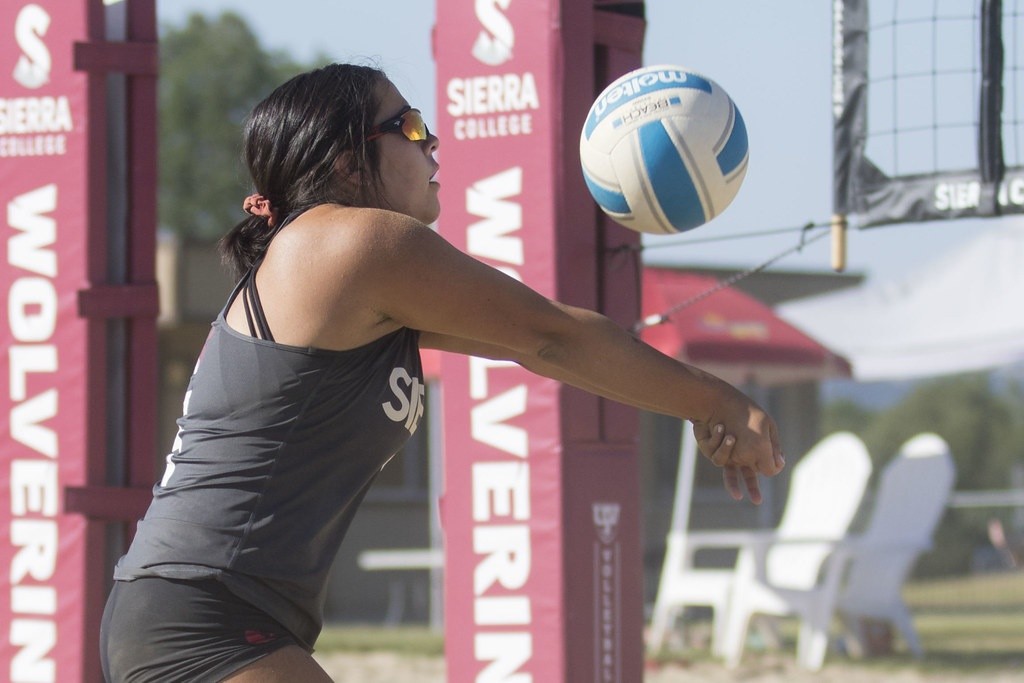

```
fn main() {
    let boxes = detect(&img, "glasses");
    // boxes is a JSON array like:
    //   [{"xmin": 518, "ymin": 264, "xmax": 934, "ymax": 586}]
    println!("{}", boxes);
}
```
[{"xmin": 359, "ymin": 107, "xmax": 431, "ymax": 142}]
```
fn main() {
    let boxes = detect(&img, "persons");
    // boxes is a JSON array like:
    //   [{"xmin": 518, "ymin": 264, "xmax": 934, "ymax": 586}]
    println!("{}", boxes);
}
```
[{"xmin": 97, "ymin": 55, "xmax": 787, "ymax": 683}]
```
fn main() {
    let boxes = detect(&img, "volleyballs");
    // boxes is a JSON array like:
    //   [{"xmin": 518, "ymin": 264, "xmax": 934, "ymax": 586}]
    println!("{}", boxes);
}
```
[{"xmin": 580, "ymin": 64, "xmax": 751, "ymax": 237}]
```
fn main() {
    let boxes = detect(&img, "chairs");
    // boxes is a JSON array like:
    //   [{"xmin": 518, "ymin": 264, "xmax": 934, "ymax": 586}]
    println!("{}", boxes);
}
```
[
  {"xmin": 805, "ymin": 431, "xmax": 958, "ymax": 673},
  {"xmin": 648, "ymin": 431, "xmax": 873, "ymax": 672}
]
